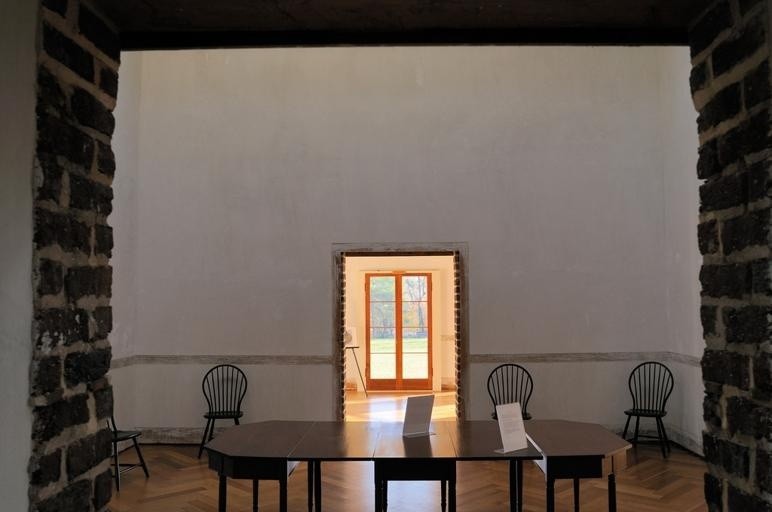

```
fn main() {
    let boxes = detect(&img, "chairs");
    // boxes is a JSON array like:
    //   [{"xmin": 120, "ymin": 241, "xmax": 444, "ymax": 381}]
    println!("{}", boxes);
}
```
[
  {"xmin": 196, "ymin": 364, "xmax": 247, "ymax": 459},
  {"xmin": 106, "ymin": 385, "xmax": 150, "ymax": 491},
  {"xmin": 487, "ymin": 364, "xmax": 533, "ymax": 420},
  {"xmin": 622, "ymin": 362, "xmax": 674, "ymax": 457}
]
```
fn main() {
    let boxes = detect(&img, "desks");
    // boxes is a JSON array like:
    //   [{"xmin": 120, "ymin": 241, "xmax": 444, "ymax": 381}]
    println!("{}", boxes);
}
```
[{"xmin": 203, "ymin": 420, "xmax": 633, "ymax": 512}]
